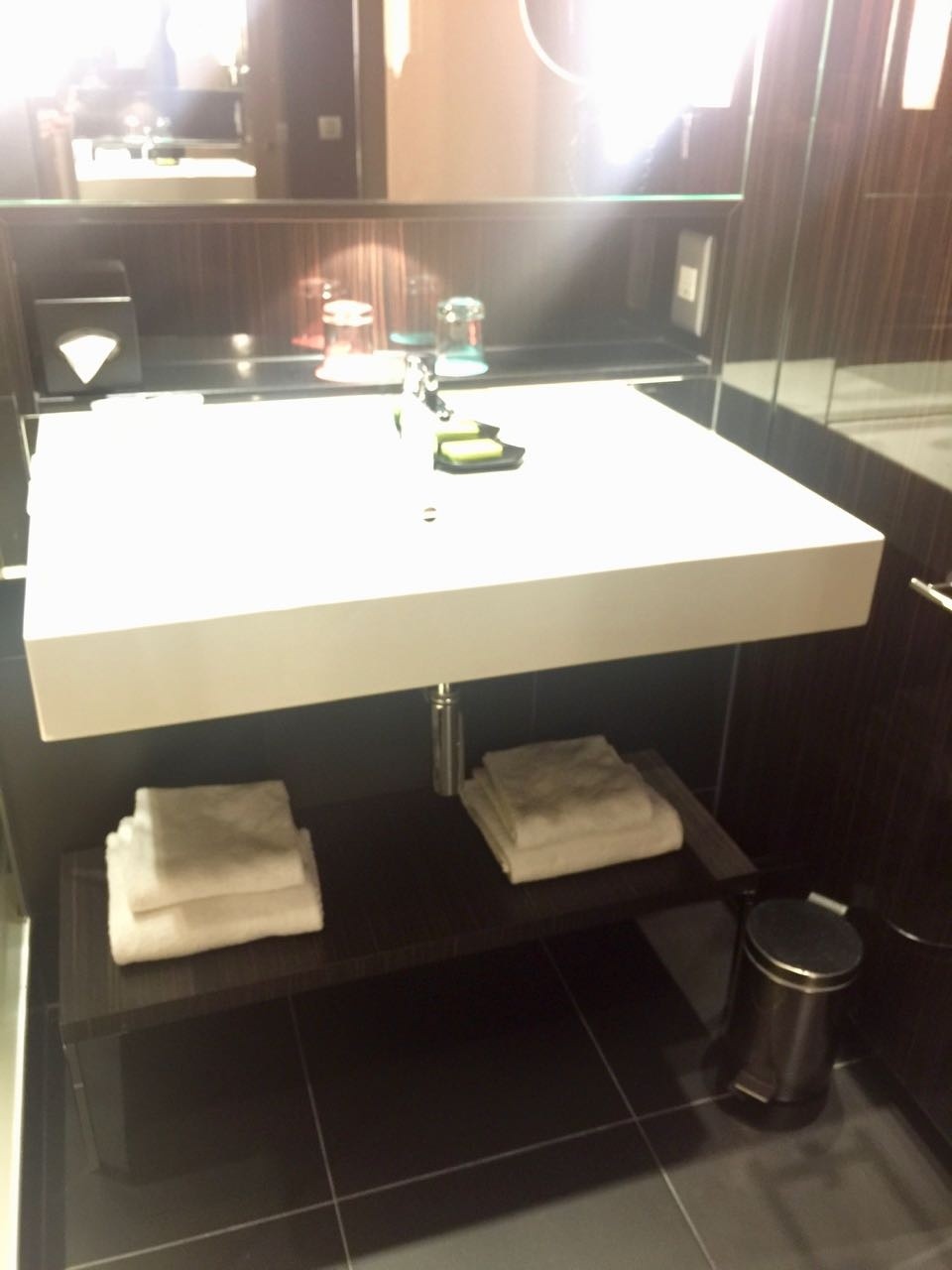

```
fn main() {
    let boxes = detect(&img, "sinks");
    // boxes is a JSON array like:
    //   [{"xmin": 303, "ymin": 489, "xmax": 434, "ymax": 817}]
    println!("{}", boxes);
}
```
[
  {"xmin": 72, "ymin": 149, "xmax": 265, "ymax": 213},
  {"xmin": 20, "ymin": 375, "xmax": 892, "ymax": 756}
]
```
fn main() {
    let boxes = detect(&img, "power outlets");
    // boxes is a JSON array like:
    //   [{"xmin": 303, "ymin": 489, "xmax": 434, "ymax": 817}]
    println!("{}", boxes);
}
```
[{"xmin": 669, "ymin": 229, "xmax": 712, "ymax": 338}]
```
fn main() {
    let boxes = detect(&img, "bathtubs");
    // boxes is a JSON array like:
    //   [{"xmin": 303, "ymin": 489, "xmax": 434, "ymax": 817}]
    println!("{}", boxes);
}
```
[{"xmin": 825, "ymin": 402, "xmax": 952, "ymax": 522}]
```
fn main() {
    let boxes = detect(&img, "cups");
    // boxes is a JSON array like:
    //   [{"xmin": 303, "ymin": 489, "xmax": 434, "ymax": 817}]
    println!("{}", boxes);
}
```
[
  {"xmin": 323, "ymin": 298, "xmax": 376, "ymax": 381},
  {"xmin": 434, "ymin": 294, "xmax": 488, "ymax": 378}
]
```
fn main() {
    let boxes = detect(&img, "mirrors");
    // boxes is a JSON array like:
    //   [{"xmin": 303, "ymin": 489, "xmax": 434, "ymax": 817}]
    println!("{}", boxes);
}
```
[{"xmin": 0, "ymin": 2, "xmax": 775, "ymax": 212}]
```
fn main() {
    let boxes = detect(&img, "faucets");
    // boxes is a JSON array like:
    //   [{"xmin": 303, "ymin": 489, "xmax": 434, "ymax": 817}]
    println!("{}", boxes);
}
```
[{"xmin": 391, "ymin": 354, "xmax": 456, "ymax": 514}]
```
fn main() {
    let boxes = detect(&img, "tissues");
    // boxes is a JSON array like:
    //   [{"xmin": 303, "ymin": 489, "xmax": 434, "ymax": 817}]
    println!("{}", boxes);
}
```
[{"xmin": 27, "ymin": 275, "xmax": 146, "ymax": 405}]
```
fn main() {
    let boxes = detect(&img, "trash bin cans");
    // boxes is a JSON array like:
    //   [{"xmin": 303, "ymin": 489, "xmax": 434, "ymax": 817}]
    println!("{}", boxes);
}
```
[{"xmin": 707, "ymin": 897, "xmax": 867, "ymax": 1134}]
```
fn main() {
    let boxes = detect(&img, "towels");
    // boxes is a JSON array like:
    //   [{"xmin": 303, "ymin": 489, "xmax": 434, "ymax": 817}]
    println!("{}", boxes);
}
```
[
  {"xmin": 98, "ymin": 820, "xmax": 327, "ymax": 969},
  {"xmin": 126, "ymin": 778, "xmax": 309, "ymax": 914},
  {"xmin": 470, "ymin": 731, "xmax": 659, "ymax": 850},
  {"xmin": 456, "ymin": 776, "xmax": 691, "ymax": 892}
]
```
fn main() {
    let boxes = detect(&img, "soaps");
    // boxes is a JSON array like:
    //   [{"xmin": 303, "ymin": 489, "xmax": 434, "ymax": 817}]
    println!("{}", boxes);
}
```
[
  {"xmin": 156, "ymin": 157, "xmax": 179, "ymax": 167},
  {"xmin": 438, "ymin": 434, "xmax": 508, "ymax": 466},
  {"xmin": 424, "ymin": 413, "xmax": 487, "ymax": 437}
]
